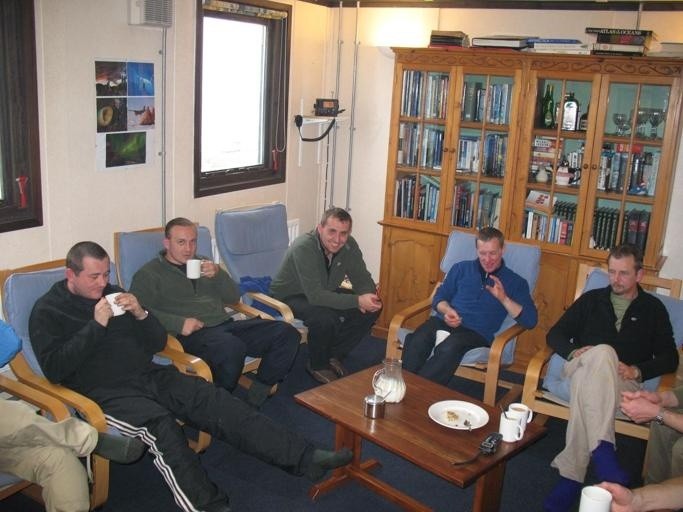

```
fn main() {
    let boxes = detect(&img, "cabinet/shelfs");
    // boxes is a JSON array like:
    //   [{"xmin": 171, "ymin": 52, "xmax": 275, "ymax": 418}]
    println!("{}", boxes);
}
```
[
  {"xmin": 371, "ymin": 222, "xmax": 448, "ymax": 339},
  {"xmin": 511, "ymin": 249, "xmax": 580, "ymax": 377},
  {"xmin": 510, "ymin": 52, "xmax": 683, "ymax": 272},
  {"xmin": 298, "ymin": 96, "xmax": 349, "ymax": 168},
  {"xmin": 377, "ymin": 47, "xmax": 529, "ymax": 242}
]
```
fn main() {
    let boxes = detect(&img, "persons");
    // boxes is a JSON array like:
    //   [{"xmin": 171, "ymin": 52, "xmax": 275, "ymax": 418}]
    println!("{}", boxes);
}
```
[
  {"xmin": 268, "ymin": 208, "xmax": 383, "ymax": 384},
  {"xmin": 401, "ymin": 227, "xmax": 539, "ymax": 387},
  {"xmin": 29, "ymin": 241, "xmax": 355, "ymax": 511},
  {"xmin": 591, "ymin": 474, "xmax": 682, "ymax": 512},
  {"xmin": 542, "ymin": 242, "xmax": 677, "ymax": 512},
  {"xmin": 0, "ymin": 316, "xmax": 145, "ymax": 512},
  {"xmin": 129, "ymin": 216, "xmax": 302, "ymax": 409},
  {"xmin": 619, "ymin": 385, "xmax": 683, "ymax": 488}
]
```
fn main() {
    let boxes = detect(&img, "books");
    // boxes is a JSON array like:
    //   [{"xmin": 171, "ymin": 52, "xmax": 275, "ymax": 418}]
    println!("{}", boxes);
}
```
[
  {"xmin": 400, "ymin": 69, "xmax": 448, "ymax": 120},
  {"xmin": 461, "ymin": 81, "xmax": 512, "ymax": 125},
  {"xmin": 528, "ymin": 135, "xmax": 583, "ymax": 187},
  {"xmin": 589, "ymin": 204, "xmax": 651, "ymax": 253},
  {"xmin": 396, "ymin": 122, "xmax": 444, "ymax": 170},
  {"xmin": 452, "ymin": 181, "xmax": 502, "ymax": 230},
  {"xmin": 456, "ymin": 133, "xmax": 508, "ymax": 178},
  {"xmin": 596, "ymin": 142, "xmax": 661, "ymax": 197},
  {"xmin": 521, "ymin": 190, "xmax": 577, "ymax": 245},
  {"xmin": 393, "ymin": 174, "xmax": 440, "ymax": 223},
  {"xmin": 428, "ymin": 27, "xmax": 683, "ymax": 58}
]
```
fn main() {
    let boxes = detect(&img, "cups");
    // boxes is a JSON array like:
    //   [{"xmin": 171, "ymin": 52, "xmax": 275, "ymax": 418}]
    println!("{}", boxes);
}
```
[
  {"xmin": 579, "ymin": 486, "xmax": 612, "ymax": 512},
  {"xmin": 498, "ymin": 412, "xmax": 524, "ymax": 443},
  {"xmin": 105, "ymin": 292, "xmax": 126, "ymax": 317},
  {"xmin": 509, "ymin": 403, "xmax": 533, "ymax": 432},
  {"xmin": 186, "ymin": 260, "xmax": 205, "ymax": 280},
  {"xmin": 365, "ymin": 394, "xmax": 385, "ymax": 419}
]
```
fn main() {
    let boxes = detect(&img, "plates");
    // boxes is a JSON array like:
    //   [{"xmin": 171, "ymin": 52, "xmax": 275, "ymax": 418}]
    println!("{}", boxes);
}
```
[{"xmin": 427, "ymin": 399, "xmax": 489, "ymax": 430}]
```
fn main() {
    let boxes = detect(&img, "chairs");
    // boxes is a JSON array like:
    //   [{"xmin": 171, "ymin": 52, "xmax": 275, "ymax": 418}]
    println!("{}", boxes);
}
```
[
  {"xmin": 217, "ymin": 202, "xmax": 370, "ymax": 340},
  {"xmin": 114, "ymin": 223, "xmax": 279, "ymax": 397},
  {"xmin": 386, "ymin": 229, "xmax": 542, "ymax": 412},
  {"xmin": 0, "ymin": 320, "xmax": 69, "ymax": 512},
  {"xmin": 522, "ymin": 263, "xmax": 681, "ymax": 479},
  {"xmin": 0, "ymin": 257, "xmax": 215, "ymax": 509}
]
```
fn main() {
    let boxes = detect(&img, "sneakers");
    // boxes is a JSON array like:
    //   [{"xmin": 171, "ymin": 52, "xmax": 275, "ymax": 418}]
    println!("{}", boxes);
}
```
[
  {"xmin": 305, "ymin": 358, "xmax": 337, "ymax": 384},
  {"xmin": 312, "ymin": 448, "xmax": 353, "ymax": 481},
  {"xmin": 328, "ymin": 357, "xmax": 351, "ymax": 376}
]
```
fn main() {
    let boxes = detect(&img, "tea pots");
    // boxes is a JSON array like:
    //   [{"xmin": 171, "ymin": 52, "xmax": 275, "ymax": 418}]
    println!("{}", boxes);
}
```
[{"xmin": 372, "ymin": 357, "xmax": 406, "ymax": 403}]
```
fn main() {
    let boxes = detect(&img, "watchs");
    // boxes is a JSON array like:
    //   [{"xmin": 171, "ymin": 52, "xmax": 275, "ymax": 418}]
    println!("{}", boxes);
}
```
[{"xmin": 654, "ymin": 407, "xmax": 669, "ymax": 426}]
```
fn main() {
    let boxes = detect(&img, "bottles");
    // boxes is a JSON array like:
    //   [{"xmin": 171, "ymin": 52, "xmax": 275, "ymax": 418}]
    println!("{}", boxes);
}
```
[
  {"xmin": 560, "ymin": 92, "xmax": 580, "ymax": 132},
  {"xmin": 542, "ymin": 84, "xmax": 554, "ymax": 130},
  {"xmin": 579, "ymin": 96, "xmax": 592, "ymax": 132}
]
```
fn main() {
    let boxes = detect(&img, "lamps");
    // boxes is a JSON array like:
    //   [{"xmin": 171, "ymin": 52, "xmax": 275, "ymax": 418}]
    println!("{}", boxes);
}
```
[{"xmin": 369, "ymin": 9, "xmax": 422, "ymax": 49}]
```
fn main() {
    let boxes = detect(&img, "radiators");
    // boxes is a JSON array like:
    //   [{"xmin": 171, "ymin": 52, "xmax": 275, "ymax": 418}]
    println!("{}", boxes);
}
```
[
  {"xmin": 128, "ymin": 97, "xmax": 157, "ymax": 129},
  {"xmin": 212, "ymin": 219, "xmax": 300, "ymax": 265}
]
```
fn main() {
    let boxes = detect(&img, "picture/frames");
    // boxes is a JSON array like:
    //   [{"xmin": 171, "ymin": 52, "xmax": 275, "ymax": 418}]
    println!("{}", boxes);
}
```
[
  {"xmin": 96, "ymin": 98, "xmax": 128, "ymax": 133},
  {"xmin": 94, "ymin": 60, "xmax": 128, "ymax": 95},
  {"xmin": 128, "ymin": 60, "xmax": 155, "ymax": 97},
  {"xmin": 97, "ymin": 132, "xmax": 154, "ymax": 170}
]
